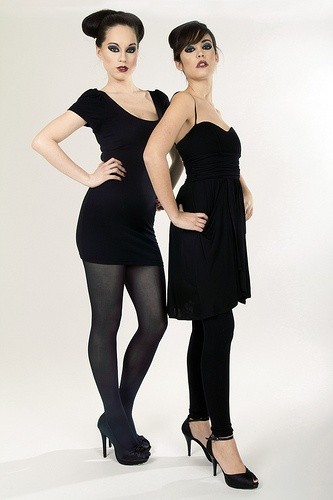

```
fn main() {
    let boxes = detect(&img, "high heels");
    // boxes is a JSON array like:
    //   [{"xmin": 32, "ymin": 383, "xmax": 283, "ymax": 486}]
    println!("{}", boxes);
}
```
[
  {"xmin": 96, "ymin": 412, "xmax": 151, "ymax": 465},
  {"xmin": 205, "ymin": 434, "xmax": 258, "ymax": 489},
  {"xmin": 181, "ymin": 416, "xmax": 213, "ymax": 463}
]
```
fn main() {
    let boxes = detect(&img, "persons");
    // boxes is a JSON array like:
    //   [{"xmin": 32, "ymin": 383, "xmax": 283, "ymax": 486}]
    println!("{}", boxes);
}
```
[
  {"xmin": 143, "ymin": 21, "xmax": 259, "ymax": 489},
  {"xmin": 32, "ymin": 9, "xmax": 185, "ymax": 465}
]
